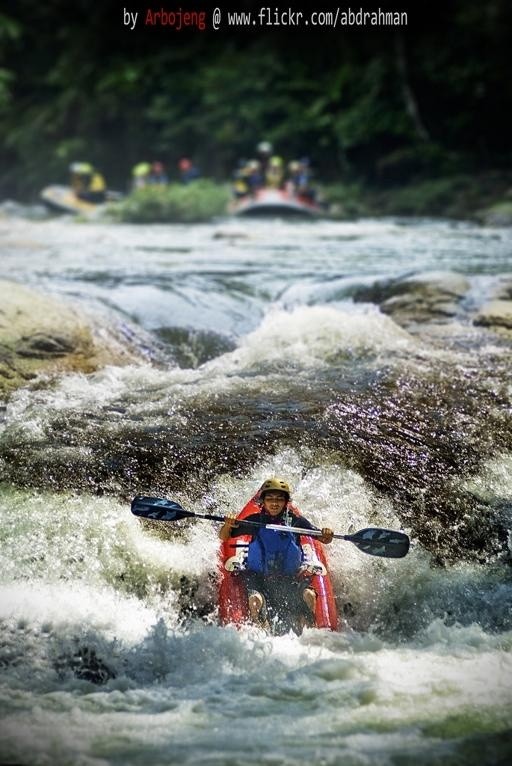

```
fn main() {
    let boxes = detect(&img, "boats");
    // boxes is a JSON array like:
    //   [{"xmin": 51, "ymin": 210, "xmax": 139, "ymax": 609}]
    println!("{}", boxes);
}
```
[
  {"xmin": 229, "ymin": 187, "xmax": 318, "ymax": 219},
  {"xmin": 39, "ymin": 184, "xmax": 121, "ymax": 214},
  {"xmin": 219, "ymin": 492, "xmax": 338, "ymax": 631}
]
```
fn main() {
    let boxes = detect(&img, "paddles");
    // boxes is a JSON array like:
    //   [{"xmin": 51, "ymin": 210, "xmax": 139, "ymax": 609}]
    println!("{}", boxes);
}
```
[{"xmin": 131, "ymin": 496, "xmax": 410, "ymax": 559}]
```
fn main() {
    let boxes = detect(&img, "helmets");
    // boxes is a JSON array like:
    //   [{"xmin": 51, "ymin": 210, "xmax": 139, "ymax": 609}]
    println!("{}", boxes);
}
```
[{"xmin": 258, "ymin": 478, "xmax": 291, "ymax": 500}]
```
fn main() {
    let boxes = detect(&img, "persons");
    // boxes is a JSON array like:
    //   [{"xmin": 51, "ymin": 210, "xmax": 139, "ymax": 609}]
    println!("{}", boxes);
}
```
[
  {"xmin": 228, "ymin": 140, "xmax": 318, "ymax": 202},
  {"xmin": 130, "ymin": 155, "xmax": 201, "ymax": 205},
  {"xmin": 67, "ymin": 160, "xmax": 107, "ymax": 204},
  {"xmin": 218, "ymin": 477, "xmax": 333, "ymax": 639}
]
